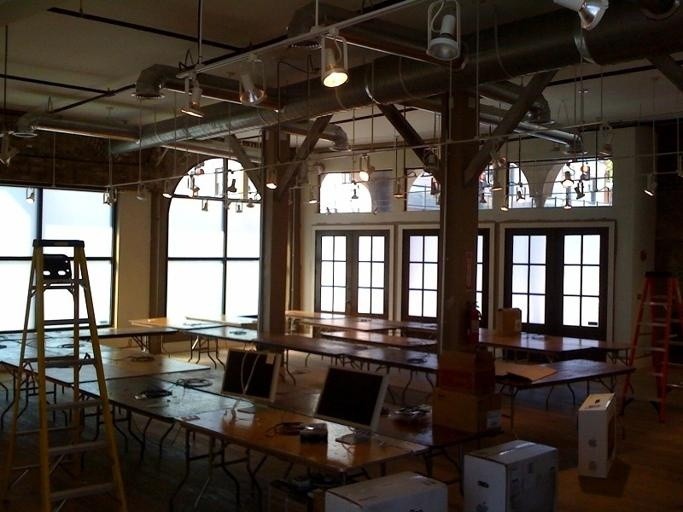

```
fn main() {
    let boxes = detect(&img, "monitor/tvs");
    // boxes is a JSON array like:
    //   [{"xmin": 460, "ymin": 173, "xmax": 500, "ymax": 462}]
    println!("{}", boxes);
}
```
[
  {"xmin": 313, "ymin": 366, "xmax": 390, "ymax": 444},
  {"xmin": 220, "ymin": 348, "xmax": 283, "ymax": 414}
]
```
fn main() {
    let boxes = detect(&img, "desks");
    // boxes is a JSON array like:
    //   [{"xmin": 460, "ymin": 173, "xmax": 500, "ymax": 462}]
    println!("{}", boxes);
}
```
[
  {"xmin": 55, "ymin": 375, "xmax": 257, "ymax": 512},
  {"xmin": 284, "ymin": 307, "xmax": 635, "ymax": 356},
  {"xmin": 184, "ymin": 326, "xmax": 286, "ymax": 384},
  {"xmin": 495, "ymin": 359, "xmax": 637, "ymax": 430},
  {"xmin": 0, "ymin": 338, "xmax": 211, "ymax": 385},
  {"xmin": 347, "ymin": 347, "xmax": 438, "ymax": 405},
  {"xmin": 252, "ymin": 333, "xmax": 368, "ymax": 384},
  {"xmin": 0, "ymin": 327, "xmax": 179, "ymax": 353},
  {"xmin": 170, "ymin": 406, "xmax": 433, "ymax": 512}
]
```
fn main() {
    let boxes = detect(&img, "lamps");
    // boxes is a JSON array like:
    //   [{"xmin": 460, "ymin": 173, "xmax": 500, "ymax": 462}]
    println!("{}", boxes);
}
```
[
  {"xmin": 600, "ymin": 144, "xmax": 612, "ymax": 156},
  {"xmin": 516, "ymin": 184, "xmax": 526, "ymax": 204},
  {"xmin": 394, "ymin": 184, "xmax": 404, "ymax": 198},
  {"xmin": 359, "ymin": 155, "xmax": 376, "ymax": 181},
  {"xmin": 321, "ymin": 36, "xmax": 349, "ymax": 88},
  {"xmin": 266, "ymin": 317, "xmax": 278, "ymax": 333},
  {"xmin": 186, "ymin": 177, "xmax": 200, "ymax": 198},
  {"xmin": 561, "ymin": 171, "xmax": 573, "ymax": 188},
  {"xmin": 484, "ymin": 140, "xmax": 506, "ymax": 170},
  {"xmin": 553, "ymin": 0, "xmax": 609, "ymax": 31},
  {"xmin": 136, "ymin": 182, "xmax": 145, "ymax": 200},
  {"xmin": 430, "ymin": 182, "xmax": 438, "ymax": 195},
  {"xmin": 480, "ymin": 193, "xmax": 487, "ymax": 205},
  {"xmin": 491, "ymin": 181, "xmax": 504, "ymax": 192},
  {"xmin": 180, "ymin": 77, "xmax": 205, "ymax": 118},
  {"xmin": 644, "ymin": 175, "xmax": 658, "ymax": 196},
  {"xmin": 574, "ymin": 182, "xmax": 585, "ymax": 199},
  {"xmin": 103, "ymin": 188, "xmax": 117, "ymax": 205},
  {"xmin": 501, "ymin": 199, "xmax": 509, "ymax": 211},
  {"xmin": 202, "ymin": 198, "xmax": 209, "ymax": 213},
  {"xmin": 308, "ymin": 192, "xmax": 316, "ymax": 205},
  {"xmin": 247, "ymin": 198, "xmax": 254, "ymax": 209},
  {"xmin": 428, "ymin": 1, "xmax": 460, "ymax": 62},
  {"xmin": 237, "ymin": 205, "xmax": 242, "ymax": 214},
  {"xmin": 424, "ymin": 154, "xmax": 438, "ymax": 174},
  {"xmin": 352, "ymin": 189, "xmax": 359, "ymax": 200},
  {"xmin": 26, "ymin": 187, "xmax": 39, "ymax": 204},
  {"xmin": 228, "ymin": 179, "xmax": 237, "ymax": 192},
  {"xmin": 163, "ymin": 178, "xmax": 173, "ymax": 199},
  {"xmin": 239, "ymin": 71, "xmax": 265, "ymax": 104},
  {"xmin": 563, "ymin": 199, "xmax": 572, "ymax": 210}
]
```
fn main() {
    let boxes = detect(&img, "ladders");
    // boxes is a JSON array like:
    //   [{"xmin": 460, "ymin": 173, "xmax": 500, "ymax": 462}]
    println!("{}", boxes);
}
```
[
  {"xmin": 620, "ymin": 269, "xmax": 683, "ymax": 423},
  {"xmin": 0, "ymin": 239, "xmax": 127, "ymax": 512}
]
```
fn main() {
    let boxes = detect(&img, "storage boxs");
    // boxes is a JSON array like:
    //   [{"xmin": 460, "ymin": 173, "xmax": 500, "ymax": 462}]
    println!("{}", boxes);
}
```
[
  {"xmin": 464, "ymin": 440, "xmax": 558, "ymax": 511},
  {"xmin": 325, "ymin": 471, "xmax": 448, "ymax": 512}
]
